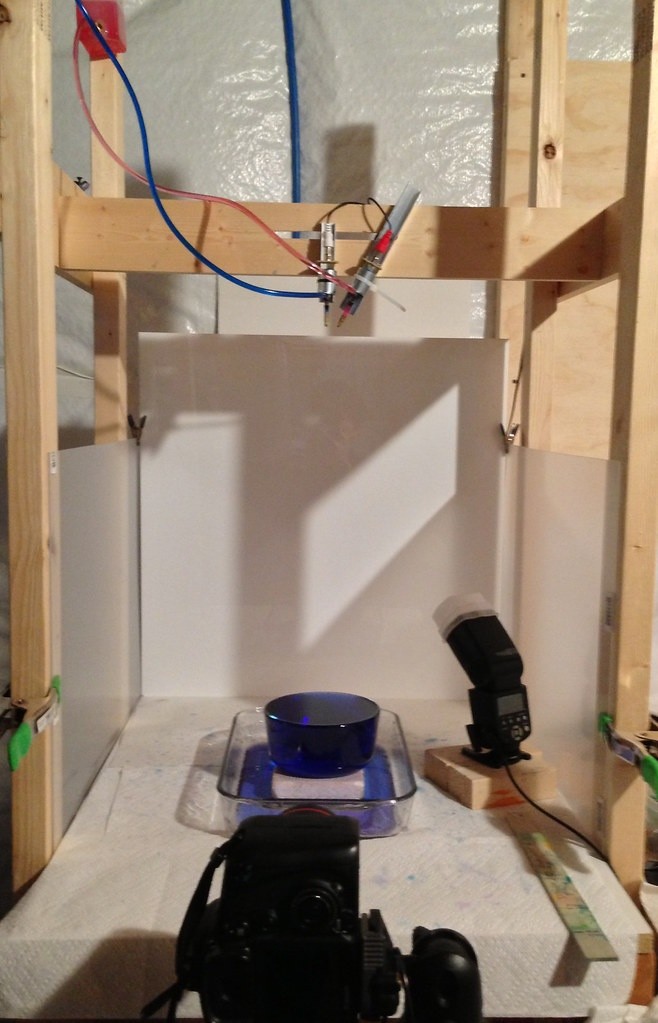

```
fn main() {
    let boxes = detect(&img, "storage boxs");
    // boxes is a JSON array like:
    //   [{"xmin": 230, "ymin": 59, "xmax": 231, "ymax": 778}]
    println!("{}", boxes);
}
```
[{"xmin": 215, "ymin": 707, "xmax": 417, "ymax": 839}]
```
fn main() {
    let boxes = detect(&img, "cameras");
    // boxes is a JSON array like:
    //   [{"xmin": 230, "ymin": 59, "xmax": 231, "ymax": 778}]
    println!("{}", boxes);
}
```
[{"xmin": 174, "ymin": 803, "xmax": 402, "ymax": 1022}]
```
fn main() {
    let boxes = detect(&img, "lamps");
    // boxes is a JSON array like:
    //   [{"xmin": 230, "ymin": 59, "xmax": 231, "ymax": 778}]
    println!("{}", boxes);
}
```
[{"xmin": 432, "ymin": 591, "xmax": 533, "ymax": 769}]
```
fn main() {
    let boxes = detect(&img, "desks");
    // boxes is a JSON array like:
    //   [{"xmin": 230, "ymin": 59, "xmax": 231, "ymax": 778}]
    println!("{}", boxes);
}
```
[{"xmin": 0, "ymin": 695, "xmax": 658, "ymax": 1023}]
